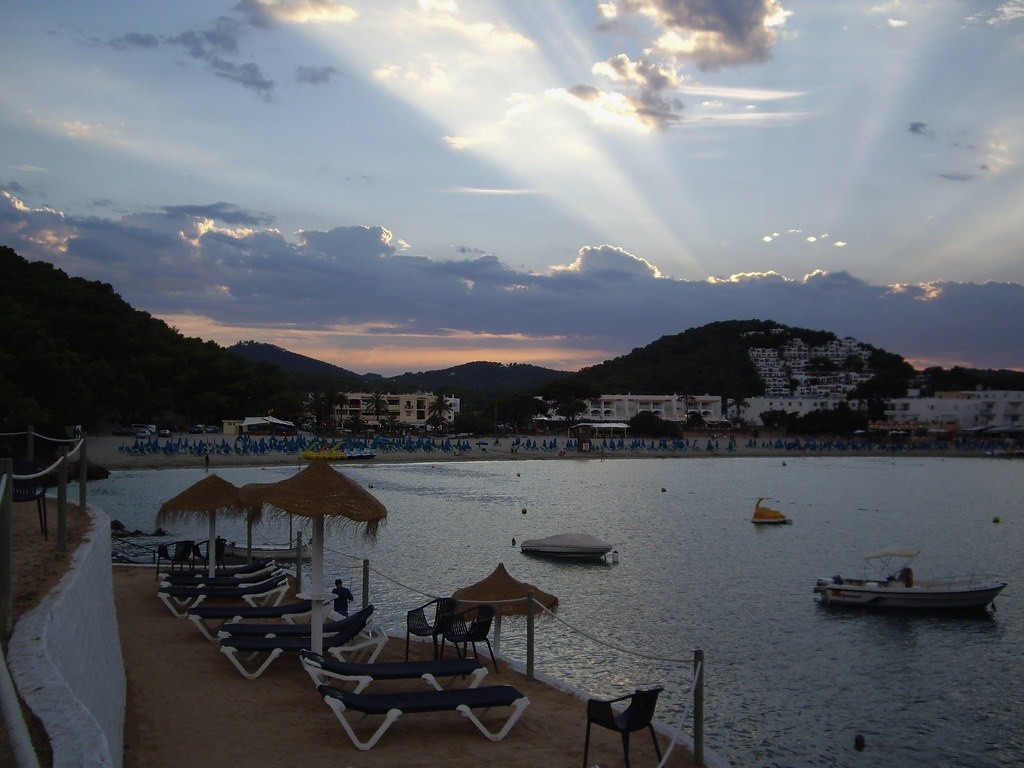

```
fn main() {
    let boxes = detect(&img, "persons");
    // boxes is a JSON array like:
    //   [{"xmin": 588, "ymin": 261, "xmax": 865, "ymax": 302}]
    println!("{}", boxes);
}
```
[
  {"xmin": 833, "ymin": 574, "xmax": 845, "ymax": 585},
  {"xmin": 331, "ymin": 579, "xmax": 354, "ymax": 619},
  {"xmin": 560, "ymin": 448, "xmax": 567, "ymax": 456},
  {"xmin": 511, "ymin": 444, "xmax": 518, "ymax": 455},
  {"xmin": 204, "ymin": 452, "xmax": 209, "ymax": 471}
]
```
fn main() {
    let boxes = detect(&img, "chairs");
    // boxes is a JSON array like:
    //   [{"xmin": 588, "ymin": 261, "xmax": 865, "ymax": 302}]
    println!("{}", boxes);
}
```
[
  {"xmin": 440, "ymin": 603, "xmax": 499, "ymax": 680},
  {"xmin": 220, "ymin": 609, "xmax": 389, "ymax": 680},
  {"xmin": 297, "ymin": 649, "xmax": 489, "ymax": 694},
  {"xmin": 156, "ymin": 540, "xmax": 195, "ymax": 582},
  {"xmin": 583, "ymin": 687, "xmax": 665, "ymax": 768},
  {"xmin": 157, "ymin": 556, "xmax": 289, "ymax": 619},
  {"xmin": 12, "ymin": 458, "xmax": 49, "ymax": 541},
  {"xmin": 186, "ymin": 593, "xmax": 338, "ymax": 643},
  {"xmin": 216, "ymin": 604, "xmax": 377, "ymax": 662},
  {"xmin": 405, "ymin": 597, "xmax": 462, "ymax": 662},
  {"xmin": 192, "ymin": 537, "xmax": 227, "ymax": 571},
  {"xmin": 318, "ymin": 684, "xmax": 531, "ymax": 751}
]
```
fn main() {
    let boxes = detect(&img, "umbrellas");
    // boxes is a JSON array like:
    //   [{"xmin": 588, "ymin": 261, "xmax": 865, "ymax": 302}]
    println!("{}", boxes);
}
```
[
  {"xmin": 155, "ymin": 473, "xmax": 263, "ymax": 579},
  {"xmin": 452, "ymin": 563, "xmax": 559, "ymax": 654},
  {"xmin": 245, "ymin": 458, "xmax": 387, "ymax": 655}
]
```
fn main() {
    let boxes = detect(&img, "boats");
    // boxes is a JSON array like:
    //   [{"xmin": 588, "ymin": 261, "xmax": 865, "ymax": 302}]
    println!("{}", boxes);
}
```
[
  {"xmin": 813, "ymin": 548, "xmax": 1007, "ymax": 607},
  {"xmin": 751, "ymin": 497, "xmax": 793, "ymax": 526},
  {"xmin": 520, "ymin": 532, "xmax": 614, "ymax": 559}
]
